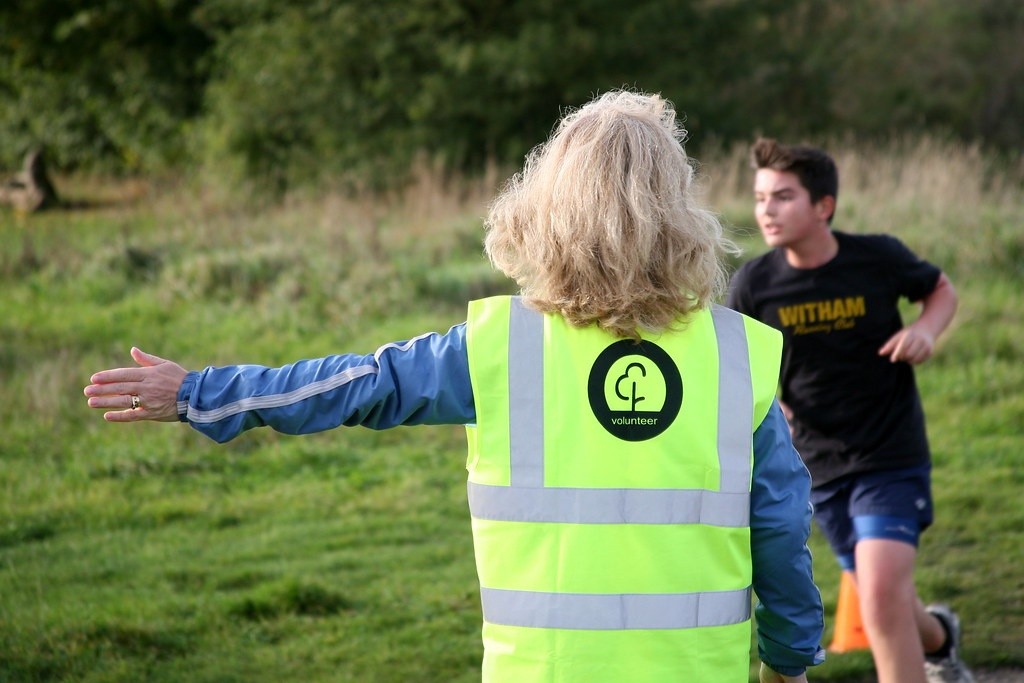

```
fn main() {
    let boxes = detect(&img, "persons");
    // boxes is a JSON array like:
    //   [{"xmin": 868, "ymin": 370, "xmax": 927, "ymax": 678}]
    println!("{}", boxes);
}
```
[
  {"xmin": 726, "ymin": 136, "xmax": 974, "ymax": 683},
  {"xmin": 81, "ymin": 81, "xmax": 833, "ymax": 681}
]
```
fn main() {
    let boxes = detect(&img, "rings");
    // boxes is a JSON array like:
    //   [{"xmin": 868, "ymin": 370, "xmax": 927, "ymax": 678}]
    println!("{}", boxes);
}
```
[{"xmin": 131, "ymin": 395, "xmax": 139, "ymax": 408}]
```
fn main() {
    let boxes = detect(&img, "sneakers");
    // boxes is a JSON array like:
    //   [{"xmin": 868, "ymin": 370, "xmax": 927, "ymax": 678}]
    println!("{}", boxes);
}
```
[{"xmin": 922, "ymin": 606, "xmax": 972, "ymax": 683}]
